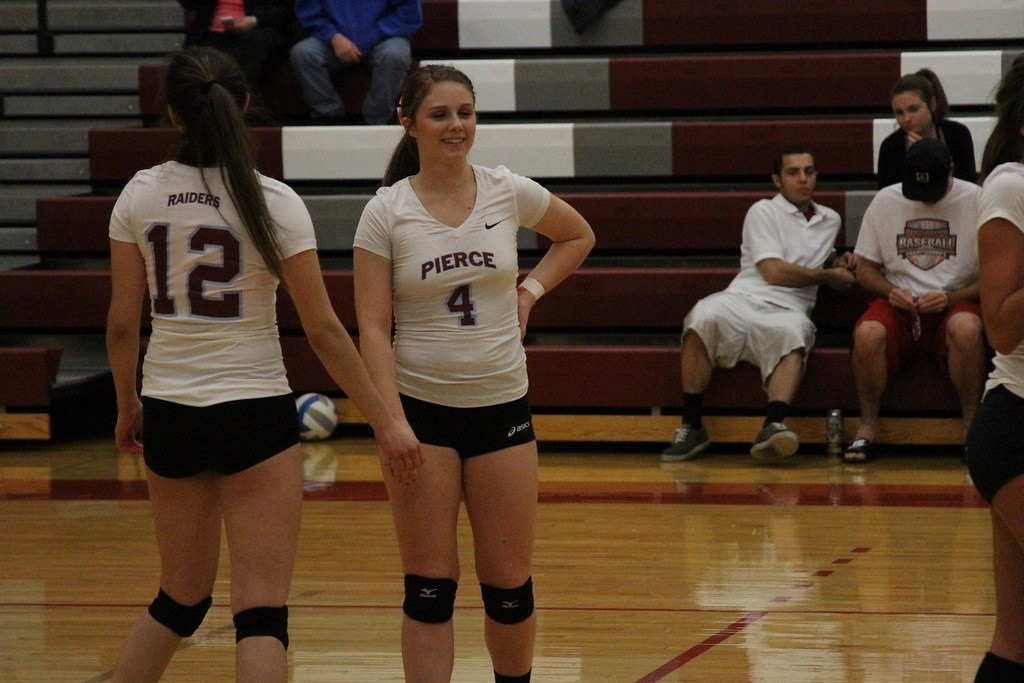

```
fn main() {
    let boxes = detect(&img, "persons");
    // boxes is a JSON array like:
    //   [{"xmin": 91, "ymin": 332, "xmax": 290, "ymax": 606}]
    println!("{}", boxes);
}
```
[
  {"xmin": 842, "ymin": 138, "xmax": 982, "ymax": 464},
  {"xmin": 966, "ymin": 52, "xmax": 1024, "ymax": 683},
  {"xmin": 289, "ymin": 0, "xmax": 424, "ymax": 126},
  {"xmin": 353, "ymin": 64, "xmax": 597, "ymax": 683},
  {"xmin": 105, "ymin": 46, "xmax": 427, "ymax": 683},
  {"xmin": 657, "ymin": 144, "xmax": 860, "ymax": 463},
  {"xmin": 876, "ymin": 68, "xmax": 977, "ymax": 190},
  {"xmin": 142, "ymin": 0, "xmax": 297, "ymax": 128}
]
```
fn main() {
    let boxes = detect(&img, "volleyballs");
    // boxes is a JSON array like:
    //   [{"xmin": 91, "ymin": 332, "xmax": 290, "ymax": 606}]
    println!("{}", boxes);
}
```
[{"xmin": 292, "ymin": 391, "xmax": 339, "ymax": 442}]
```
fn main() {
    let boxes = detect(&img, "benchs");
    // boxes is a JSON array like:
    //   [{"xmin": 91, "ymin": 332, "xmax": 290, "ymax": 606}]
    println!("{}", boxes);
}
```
[{"xmin": 0, "ymin": 0, "xmax": 1024, "ymax": 442}]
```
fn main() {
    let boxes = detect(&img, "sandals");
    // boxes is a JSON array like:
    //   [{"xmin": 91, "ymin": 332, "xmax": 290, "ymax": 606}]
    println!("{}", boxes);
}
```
[{"xmin": 842, "ymin": 438, "xmax": 880, "ymax": 463}]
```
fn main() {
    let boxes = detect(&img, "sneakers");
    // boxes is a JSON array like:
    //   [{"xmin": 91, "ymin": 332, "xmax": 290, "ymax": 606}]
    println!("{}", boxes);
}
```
[
  {"xmin": 659, "ymin": 423, "xmax": 712, "ymax": 462},
  {"xmin": 749, "ymin": 422, "xmax": 800, "ymax": 460}
]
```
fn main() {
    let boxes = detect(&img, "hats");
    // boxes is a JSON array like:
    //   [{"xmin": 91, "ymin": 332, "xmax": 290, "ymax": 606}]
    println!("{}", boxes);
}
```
[{"xmin": 902, "ymin": 137, "xmax": 953, "ymax": 201}]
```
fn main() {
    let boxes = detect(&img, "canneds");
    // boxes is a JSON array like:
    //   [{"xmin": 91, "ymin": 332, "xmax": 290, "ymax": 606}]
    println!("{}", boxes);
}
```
[{"xmin": 826, "ymin": 409, "xmax": 843, "ymax": 444}]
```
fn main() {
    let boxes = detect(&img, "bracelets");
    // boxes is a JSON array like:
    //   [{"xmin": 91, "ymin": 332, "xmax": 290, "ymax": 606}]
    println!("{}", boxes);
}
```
[{"xmin": 518, "ymin": 278, "xmax": 545, "ymax": 300}]
